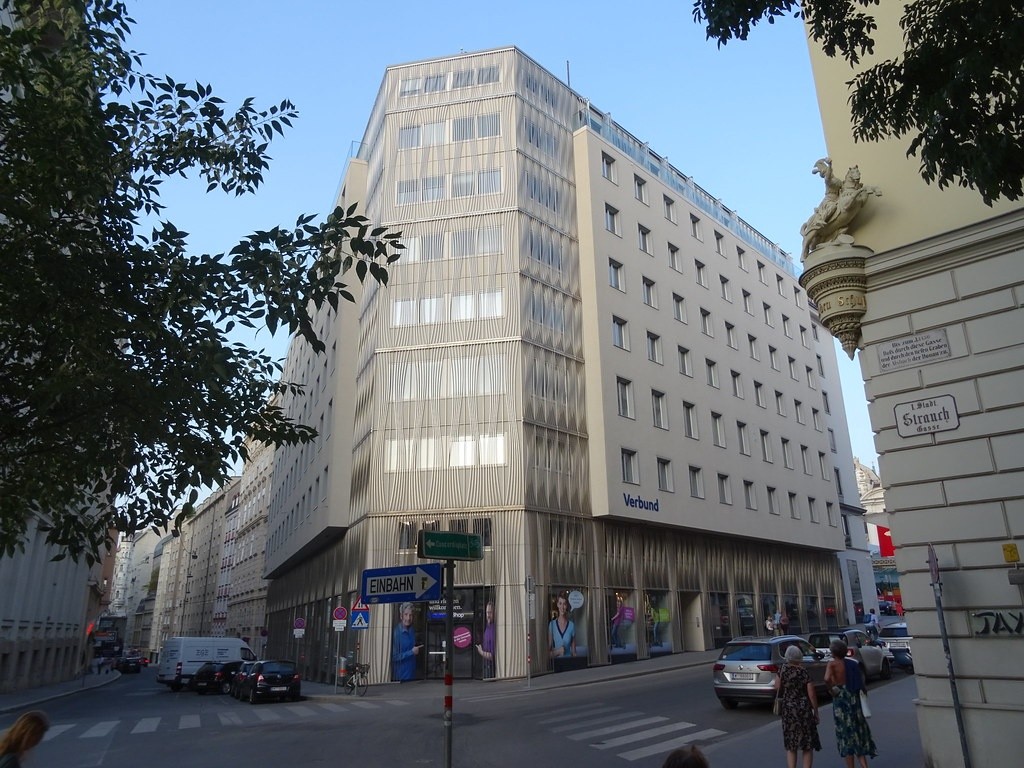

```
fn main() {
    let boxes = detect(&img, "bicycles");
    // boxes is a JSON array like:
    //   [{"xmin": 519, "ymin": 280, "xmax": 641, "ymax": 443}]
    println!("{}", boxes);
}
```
[{"xmin": 344, "ymin": 662, "xmax": 370, "ymax": 697}]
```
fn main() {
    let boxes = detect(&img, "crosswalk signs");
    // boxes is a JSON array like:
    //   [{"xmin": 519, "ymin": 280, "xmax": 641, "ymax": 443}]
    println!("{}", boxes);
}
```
[{"xmin": 352, "ymin": 608, "xmax": 369, "ymax": 629}]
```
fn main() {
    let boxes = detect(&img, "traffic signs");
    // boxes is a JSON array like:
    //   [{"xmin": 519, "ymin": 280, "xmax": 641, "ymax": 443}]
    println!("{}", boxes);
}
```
[
  {"xmin": 423, "ymin": 530, "xmax": 484, "ymax": 560},
  {"xmin": 361, "ymin": 563, "xmax": 443, "ymax": 604}
]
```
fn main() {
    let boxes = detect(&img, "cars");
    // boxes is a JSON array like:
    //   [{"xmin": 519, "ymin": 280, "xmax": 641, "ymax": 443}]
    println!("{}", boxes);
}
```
[
  {"xmin": 229, "ymin": 661, "xmax": 257, "ymax": 699},
  {"xmin": 111, "ymin": 657, "xmax": 149, "ymax": 674},
  {"xmin": 238, "ymin": 660, "xmax": 301, "ymax": 704},
  {"xmin": 879, "ymin": 623, "xmax": 914, "ymax": 675},
  {"xmin": 193, "ymin": 661, "xmax": 231, "ymax": 695},
  {"xmin": 808, "ymin": 629, "xmax": 891, "ymax": 687}
]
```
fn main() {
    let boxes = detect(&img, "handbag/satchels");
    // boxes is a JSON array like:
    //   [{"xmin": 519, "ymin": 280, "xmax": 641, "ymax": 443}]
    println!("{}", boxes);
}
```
[
  {"xmin": 861, "ymin": 691, "xmax": 872, "ymax": 718},
  {"xmin": 773, "ymin": 664, "xmax": 784, "ymax": 715}
]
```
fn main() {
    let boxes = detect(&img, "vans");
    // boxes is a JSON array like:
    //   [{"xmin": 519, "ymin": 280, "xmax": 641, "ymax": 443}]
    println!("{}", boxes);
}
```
[{"xmin": 156, "ymin": 637, "xmax": 259, "ymax": 691}]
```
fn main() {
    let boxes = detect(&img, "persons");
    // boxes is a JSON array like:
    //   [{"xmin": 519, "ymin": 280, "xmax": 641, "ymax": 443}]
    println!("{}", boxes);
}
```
[
  {"xmin": 897, "ymin": 601, "xmax": 903, "ymax": 616},
  {"xmin": 548, "ymin": 590, "xmax": 577, "ymax": 658},
  {"xmin": 97, "ymin": 655, "xmax": 103, "ymax": 675},
  {"xmin": 649, "ymin": 603, "xmax": 661, "ymax": 645},
  {"xmin": 0, "ymin": 710, "xmax": 49, "ymax": 768},
  {"xmin": 766, "ymin": 610, "xmax": 789, "ymax": 636},
  {"xmin": 776, "ymin": 645, "xmax": 821, "ymax": 768},
  {"xmin": 663, "ymin": 745, "xmax": 709, "ymax": 768},
  {"xmin": 612, "ymin": 594, "xmax": 623, "ymax": 648},
  {"xmin": 109, "ymin": 656, "xmax": 148, "ymax": 675},
  {"xmin": 865, "ymin": 609, "xmax": 881, "ymax": 640},
  {"xmin": 823, "ymin": 638, "xmax": 878, "ymax": 768},
  {"xmin": 475, "ymin": 602, "xmax": 495, "ymax": 678},
  {"xmin": 392, "ymin": 602, "xmax": 424, "ymax": 681}
]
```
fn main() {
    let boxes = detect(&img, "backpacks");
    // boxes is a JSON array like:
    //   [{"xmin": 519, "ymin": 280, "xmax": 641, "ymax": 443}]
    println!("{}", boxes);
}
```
[
  {"xmin": 769, "ymin": 623, "xmax": 774, "ymax": 628},
  {"xmin": 862, "ymin": 614, "xmax": 871, "ymax": 623}
]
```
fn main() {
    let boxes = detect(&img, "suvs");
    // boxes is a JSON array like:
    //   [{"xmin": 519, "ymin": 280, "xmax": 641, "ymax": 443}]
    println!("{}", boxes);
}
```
[{"xmin": 712, "ymin": 635, "xmax": 834, "ymax": 715}]
